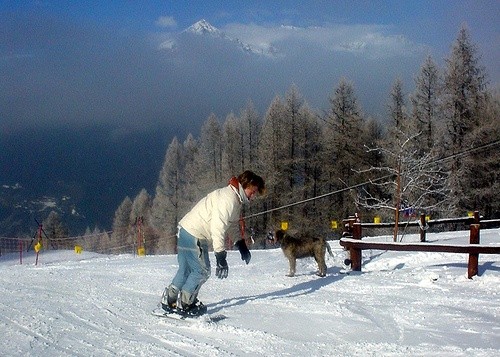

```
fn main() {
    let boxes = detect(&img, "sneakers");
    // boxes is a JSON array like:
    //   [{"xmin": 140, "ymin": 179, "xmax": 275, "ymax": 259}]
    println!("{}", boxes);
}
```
[
  {"xmin": 181, "ymin": 298, "xmax": 207, "ymax": 316},
  {"xmin": 164, "ymin": 285, "xmax": 179, "ymax": 308}
]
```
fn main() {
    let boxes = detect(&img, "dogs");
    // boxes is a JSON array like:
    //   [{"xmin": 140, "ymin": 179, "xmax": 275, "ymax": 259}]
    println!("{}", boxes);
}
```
[{"xmin": 267, "ymin": 228, "xmax": 334, "ymax": 276}]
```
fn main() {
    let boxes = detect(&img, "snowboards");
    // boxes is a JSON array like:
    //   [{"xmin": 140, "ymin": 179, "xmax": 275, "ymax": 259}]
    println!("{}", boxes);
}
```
[{"xmin": 160, "ymin": 302, "xmax": 207, "ymax": 317}]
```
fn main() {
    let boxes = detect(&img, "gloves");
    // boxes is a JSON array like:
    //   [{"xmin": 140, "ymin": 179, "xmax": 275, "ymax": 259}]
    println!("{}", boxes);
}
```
[
  {"xmin": 235, "ymin": 238, "xmax": 251, "ymax": 265},
  {"xmin": 214, "ymin": 250, "xmax": 228, "ymax": 279}
]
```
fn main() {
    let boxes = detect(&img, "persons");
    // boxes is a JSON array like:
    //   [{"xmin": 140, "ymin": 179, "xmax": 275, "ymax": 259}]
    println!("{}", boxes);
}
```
[{"xmin": 168, "ymin": 170, "xmax": 264, "ymax": 310}]
